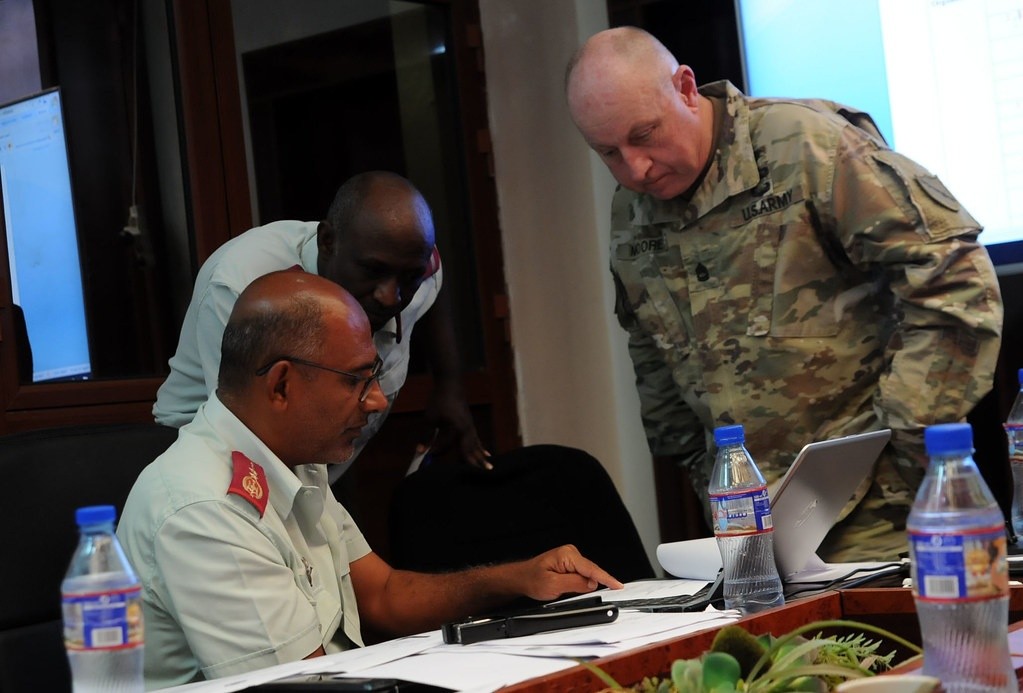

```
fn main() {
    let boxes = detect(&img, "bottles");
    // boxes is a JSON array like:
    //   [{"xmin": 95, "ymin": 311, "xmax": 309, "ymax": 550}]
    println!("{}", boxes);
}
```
[
  {"xmin": 61, "ymin": 505, "xmax": 147, "ymax": 693},
  {"xmin": 709, "ymin": 424, "xmax": 783, "ymax": 612},
  {"xmin": 904, "ymin": 421, "xmax": 1018, "ymax": 693},
  {"xmin": 1006, "ymin": 368, "xmax": 1023, "ymax": 541}
]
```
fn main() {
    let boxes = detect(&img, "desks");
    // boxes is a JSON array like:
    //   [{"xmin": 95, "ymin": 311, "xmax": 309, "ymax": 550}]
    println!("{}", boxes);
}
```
[{"xmin": 162, "ymin": 578, "xmax": 1023, "ymax": 693}]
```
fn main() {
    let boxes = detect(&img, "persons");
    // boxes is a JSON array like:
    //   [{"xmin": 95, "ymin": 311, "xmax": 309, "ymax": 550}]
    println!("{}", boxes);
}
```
[
  {"xmin": 106, "ymin": 270, "xmax": 625, "ymax": 693},
  {"xmin": 152, "ymin": 170, "xmax": 494, "ymax": 488},
  {"xmin": 564, "ymin": 25, "xmax": 1004, "ymax": 563}
]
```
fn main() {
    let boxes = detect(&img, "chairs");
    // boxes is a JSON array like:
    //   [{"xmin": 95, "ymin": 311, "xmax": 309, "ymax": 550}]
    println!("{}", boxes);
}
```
[
  {"xmin": 0, "ymin": 425, "xmax": 177, "ymax": 693},
  {"xmin": 384, "ymin": 444, "xmax": 658, "ymax": 615}
]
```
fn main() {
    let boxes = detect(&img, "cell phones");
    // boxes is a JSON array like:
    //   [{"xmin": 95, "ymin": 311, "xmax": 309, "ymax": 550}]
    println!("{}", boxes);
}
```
[{"xmin": 262, "ymin": 671, "xmax": 398, "ymax": 692}]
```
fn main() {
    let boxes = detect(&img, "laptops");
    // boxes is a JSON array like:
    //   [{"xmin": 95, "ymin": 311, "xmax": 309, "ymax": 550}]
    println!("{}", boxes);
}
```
[{"xmin": 535, "ymin": 427, "xmax": 893, "ymax": 615}]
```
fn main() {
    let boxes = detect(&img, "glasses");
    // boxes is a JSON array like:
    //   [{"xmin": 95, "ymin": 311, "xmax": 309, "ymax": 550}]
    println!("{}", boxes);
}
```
[{"xmin": 256, "ymin": 355, "xmax": 383, "ymax": 402}]
